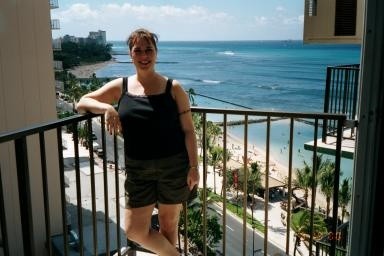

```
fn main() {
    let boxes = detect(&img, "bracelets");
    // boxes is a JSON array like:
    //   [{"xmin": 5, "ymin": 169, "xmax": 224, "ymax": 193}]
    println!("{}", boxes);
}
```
[{"xmin": 190, "ymin": 164, "xmax": 199, "ymax": 170}]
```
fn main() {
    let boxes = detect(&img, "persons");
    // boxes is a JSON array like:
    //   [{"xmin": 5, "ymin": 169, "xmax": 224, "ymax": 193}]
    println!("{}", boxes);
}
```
[{"xmin": 74, "ymin": 27, "xmax": 201, "ymax": 256}]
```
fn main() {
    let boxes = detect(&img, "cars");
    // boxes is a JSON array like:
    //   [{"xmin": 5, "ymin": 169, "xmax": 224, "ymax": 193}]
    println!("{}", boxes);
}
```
[{"xmin": 81, "ymin": 131, "xmax": 103, "ymax": 157}]
